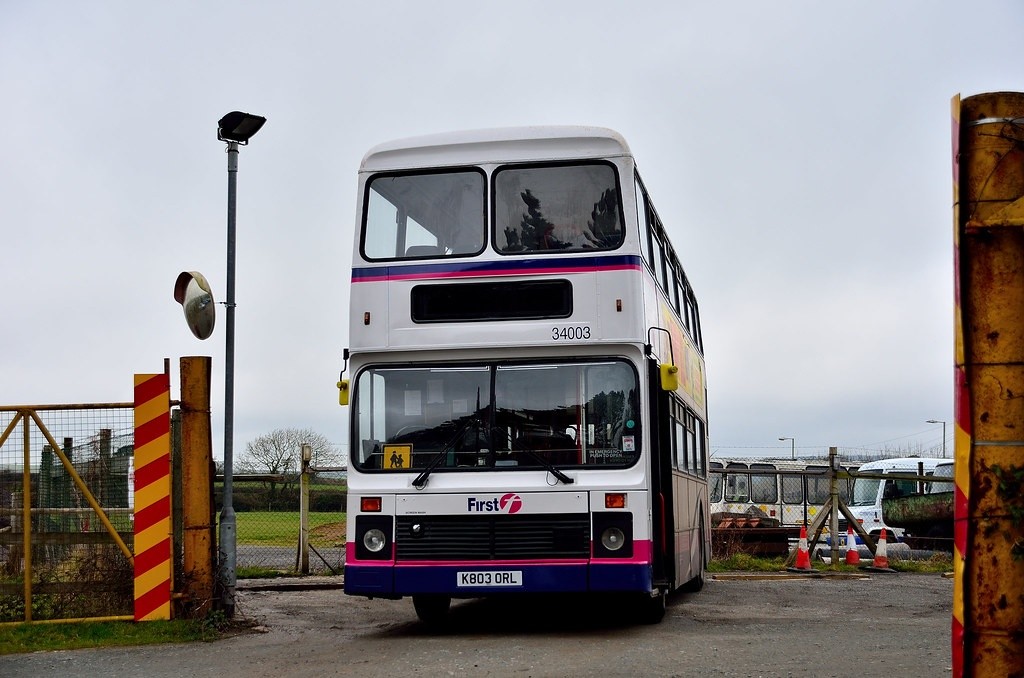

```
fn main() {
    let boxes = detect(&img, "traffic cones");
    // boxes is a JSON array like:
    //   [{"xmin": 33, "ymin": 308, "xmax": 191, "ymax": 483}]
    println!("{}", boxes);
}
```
[
  {"xmin": 845, "ymin": 522, "xmax": 863, "ymax": 566},
  {"xmin": 786, "ymin": 525, "xmax": 820, "ymax": 574},
  {"xmin": 856, "ymin": 529, "xmax": 897, "ymax": 572}
]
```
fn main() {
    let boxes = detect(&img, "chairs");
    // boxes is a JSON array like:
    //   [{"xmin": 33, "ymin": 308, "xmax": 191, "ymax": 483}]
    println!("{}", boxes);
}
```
[
  {"xmin": 393, "ymin": 419, "xmax": 554, "ymax": 460},
  {"xmin": 405, "ymin": 245, "xmax": 480, "ymax": 257}
]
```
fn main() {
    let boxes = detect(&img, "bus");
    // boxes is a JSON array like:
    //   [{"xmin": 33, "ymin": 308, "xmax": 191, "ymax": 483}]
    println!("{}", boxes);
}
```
[
  {"xmin": 704, "ymin": 454, "xmax": 868, "ymax": 542},
  {"xmin": 337, "ymin": 123, "xmax": 714, "ymax": 629},
  {"xmin": 847, "ymin": 457, "xmax": 954, "ymax": 552}
]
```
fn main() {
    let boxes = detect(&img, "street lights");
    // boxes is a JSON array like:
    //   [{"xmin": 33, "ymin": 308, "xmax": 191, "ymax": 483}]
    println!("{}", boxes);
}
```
[
  {"xmin": 925, "ymin": 419, "xmax": 946, "ymax": 459},
  {"xmin": 778, "ymin": 436, "xmax": 795, "ymax": 460},
  {"xmin": 218, "ymin": 110, "xmax": 268, "ymax": 623}
]
